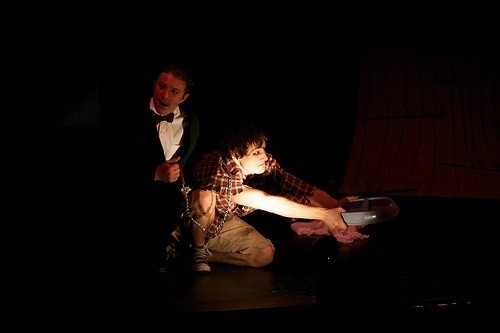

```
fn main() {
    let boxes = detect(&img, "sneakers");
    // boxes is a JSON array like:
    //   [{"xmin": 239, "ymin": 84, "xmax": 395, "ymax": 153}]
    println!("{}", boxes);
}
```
[{"xmin": 190, "ymin": 245, "xmax": 211, "ymax": 272}]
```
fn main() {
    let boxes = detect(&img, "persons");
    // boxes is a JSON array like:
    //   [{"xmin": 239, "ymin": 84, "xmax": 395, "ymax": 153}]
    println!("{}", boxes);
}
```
[
  {"xmin": 163, "ymin": 122, "xmax": 361, "ymax": 273},
  {"xmin": 102, "ymin": 59, "xmax": 200, "ymax": 279}
]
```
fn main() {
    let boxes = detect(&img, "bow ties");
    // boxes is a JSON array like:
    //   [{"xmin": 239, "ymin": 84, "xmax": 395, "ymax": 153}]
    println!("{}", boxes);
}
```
[{"xmin": 151, "ymin": 112, "xmax": 174, "ymax": 124}]
